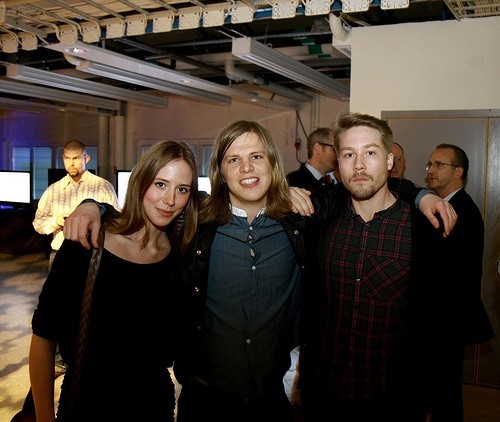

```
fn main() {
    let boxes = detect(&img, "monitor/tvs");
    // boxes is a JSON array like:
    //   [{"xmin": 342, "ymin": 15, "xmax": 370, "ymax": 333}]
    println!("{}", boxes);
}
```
[
  {"xmin": 198, "ymin": 177, "xmax": 212, "ymax": 195},
  {"xmin": 48, "ymin": 169, "xmax": 96, "ymax": 189},
  {"xmin": 0, "ymin": 169, "xmax": 33, "ymax": 208},
  {"xmin": 117, "ymin": 170, "xmax": 133, "ymax": 201}
]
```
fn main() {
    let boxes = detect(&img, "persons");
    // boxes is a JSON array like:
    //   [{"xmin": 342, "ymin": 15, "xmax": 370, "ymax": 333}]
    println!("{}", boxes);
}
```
[
  {"xmin": 29, "ymin": 140, "xmax": 199, "ymax": 422},
  {"xmin": 412, "ymin": 143, "xmax": 485, "ymax": 421},
  {"xmin": 31, "ymin": 140, "xmax": 123, "ymax": 273},
  {"xmin": 63, "ymin": 120, "xmax": 459, "ymax": 422},
  {"xmin": 284, "ymin": 126, "xmax": 338, "ymax": 190},
  {"xmin": 388, "ymin": 142, "xmax": 407, "ymax": 180},
  {"xmin": 288, "ymin": 111, "xmax": 500, "ymax": 422}
]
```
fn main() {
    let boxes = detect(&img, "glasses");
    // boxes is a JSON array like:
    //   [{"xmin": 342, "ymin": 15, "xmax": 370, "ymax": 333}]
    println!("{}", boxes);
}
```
[{"xmin": 425, "ymin": 161, "xmax": 458, "ymax": 171}]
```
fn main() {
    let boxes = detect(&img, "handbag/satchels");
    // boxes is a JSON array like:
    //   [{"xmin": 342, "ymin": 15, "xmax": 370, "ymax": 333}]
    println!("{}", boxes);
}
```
[{"xmin": 10, "ymin": 387, "xmax": 36, "ymax": 422}]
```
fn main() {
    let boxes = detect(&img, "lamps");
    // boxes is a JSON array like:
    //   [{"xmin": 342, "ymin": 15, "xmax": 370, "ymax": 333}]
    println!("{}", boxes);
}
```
[{"xmin": 0, "ymin": 29, "xmax": 350, "ymax": 111}]
